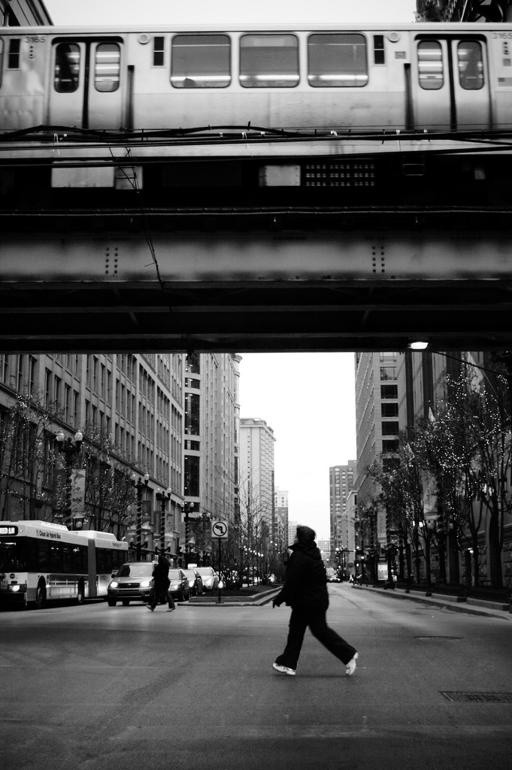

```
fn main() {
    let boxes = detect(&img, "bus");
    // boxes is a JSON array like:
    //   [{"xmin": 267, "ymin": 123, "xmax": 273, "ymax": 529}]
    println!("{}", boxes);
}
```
[{"xmin": 0, "ymin": 520, "xmax": 129, "ymax": 609}]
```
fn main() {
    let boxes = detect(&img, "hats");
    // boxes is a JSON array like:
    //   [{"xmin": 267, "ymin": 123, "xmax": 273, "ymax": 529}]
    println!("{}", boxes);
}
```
[{"xmin": 297, "ymin": 526, "xmax": 315, "ymax": 542}]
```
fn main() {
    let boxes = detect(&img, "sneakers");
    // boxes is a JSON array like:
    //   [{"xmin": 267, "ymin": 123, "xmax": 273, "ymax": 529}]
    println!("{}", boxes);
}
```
[
  {"xmin": 345, "ymin": 651, "xmax": 359, "ymax": 677},
  {"xmin": 146, "ymin": 605, "xmax": 154, "ymax": 611},
  {"xmin": 166, "ymin": 606, "xmax": 175, "ymax": 612},
  {"xmin": 272, "ymin": 663, "xmax": 296, "ymax": 676}
]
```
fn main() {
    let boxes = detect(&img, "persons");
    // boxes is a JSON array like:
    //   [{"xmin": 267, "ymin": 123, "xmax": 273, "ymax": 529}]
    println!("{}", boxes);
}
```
[
  {"xmin": 142, "ymin": 555, "xmax": 176, "ymax": 614},
  {"xmin": 268, "ymin": 523, "xmax": 359, "ymax": 678}
]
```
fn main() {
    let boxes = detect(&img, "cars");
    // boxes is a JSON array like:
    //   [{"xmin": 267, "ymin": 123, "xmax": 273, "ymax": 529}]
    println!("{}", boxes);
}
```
[
  {"xmin": 351, "ymin": 574, "xmax": 368, "ymax": 584},
  {"xmin": 107, "ymin": 562, "xmax": 277, "ymax": 606}
]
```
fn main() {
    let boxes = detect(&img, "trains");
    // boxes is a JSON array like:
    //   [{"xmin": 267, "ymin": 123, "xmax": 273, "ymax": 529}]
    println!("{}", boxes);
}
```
[{"xmin": 0, "ymin": 22, "xmax": 512, "ymax": 133}]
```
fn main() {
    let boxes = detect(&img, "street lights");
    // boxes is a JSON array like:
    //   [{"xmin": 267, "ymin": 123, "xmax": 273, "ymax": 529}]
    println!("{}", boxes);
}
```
[
  {"xmin": 196, "ymin": 511, "xmax": 211, "ymax": 566},
  {"xmin": 238, "ymin": 545, "xmax": 264, "ymax": 567},
  {"xmin": 156, "ymin": 487, "xmax": 172, "ymax": 557},
  {"xmin": 180, "ymin": 501, "xmax": 194, "ymax": 570},
  {"xmin": 408, "ymin": 341, "xmax": 512, "ymax": 614},
  {"xmin": 129, "ymin": 471, "xmax": 150, "ymax": 562},
  {"xmin": 55, "ymin": 428, "xmax": 83, "ymax": 531}
]
